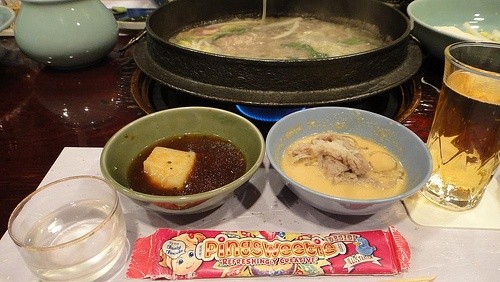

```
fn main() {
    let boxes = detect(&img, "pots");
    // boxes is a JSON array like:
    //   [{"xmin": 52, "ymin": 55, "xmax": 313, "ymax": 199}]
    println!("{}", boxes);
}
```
[{"xmin": 130, "ymin": 0, "xmax": 425, "ymax": 106}]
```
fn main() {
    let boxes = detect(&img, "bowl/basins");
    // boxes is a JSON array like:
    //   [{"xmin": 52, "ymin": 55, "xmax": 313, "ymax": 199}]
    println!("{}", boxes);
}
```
[
  {"xmin": 99, "ymin": 107, "xmax": 265, "ymax": 215},
  {"xmin": 7, "ymin": 175, "xmax": 130, "ymax": 282},
  {"xmin": 265, "ymin": 107, "xmax": 433, "ymax": 216},
  {"xmin": 406, "ymin": 0, "xmax": 500, "ymax": 62}
]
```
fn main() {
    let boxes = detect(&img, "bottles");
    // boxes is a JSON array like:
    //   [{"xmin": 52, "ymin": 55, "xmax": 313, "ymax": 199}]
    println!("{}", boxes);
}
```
[{"xmin": 13, "ymin": 0, "xmax": 118, "ymax": 74}]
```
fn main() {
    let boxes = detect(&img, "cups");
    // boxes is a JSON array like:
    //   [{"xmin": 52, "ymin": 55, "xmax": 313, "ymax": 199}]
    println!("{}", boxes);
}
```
[{"xmin": 419, "ymin": 41, "xmax": 500, "ymax": 212}]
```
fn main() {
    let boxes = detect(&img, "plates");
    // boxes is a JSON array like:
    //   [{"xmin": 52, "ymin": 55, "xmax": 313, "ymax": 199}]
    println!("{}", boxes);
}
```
[{"xmin": 0, "ymin": 4, "xmax": 15, "ymax": 36}]
